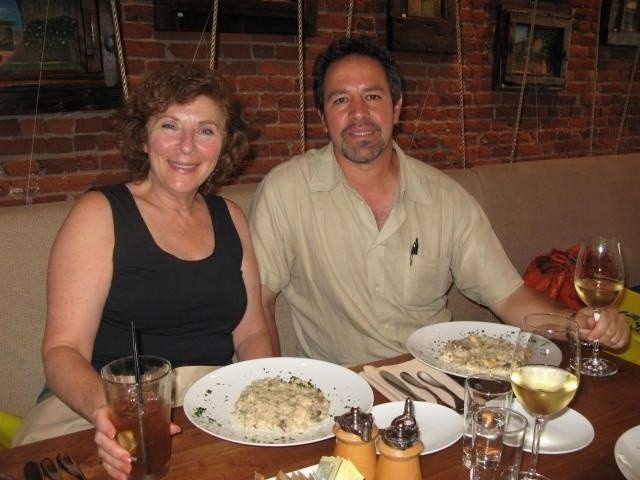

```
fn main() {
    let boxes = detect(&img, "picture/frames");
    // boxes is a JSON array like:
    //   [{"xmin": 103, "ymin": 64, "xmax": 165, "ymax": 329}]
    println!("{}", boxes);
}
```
[
  {"xmin": 502, "ymin": 12, "xmax": 572, "ymax": 87},
  {"xmin": 0, "ymin": 0, "xmax": 130, "ymax": 115},
  {"xmin": 386, "ymin": 0, "xmax": 464, "ymax": 54},
  {"xmin": 600, "ymin": 0, "xmax": 640, "ymax": 48},
  {"xmin": 153, "ymin": 0, "xmax": 317, "ymax": 37}
]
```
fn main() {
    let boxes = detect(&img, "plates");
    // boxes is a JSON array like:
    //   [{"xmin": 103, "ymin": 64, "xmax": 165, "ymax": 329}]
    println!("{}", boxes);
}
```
[
  {"xmin": 182, "ymin": 356, "xmax": 374, "ymax": 448},
  {"xmin": 614, "ymin": 424, "xmax": 640, "ymax": 480},
  {"xmin": 465, "ymin": 396, "xmax": 598, "ymax": 453},
  {"xmin": 364, "ymin": 400, "xmax": 463, "ymax": 453},
  {"xmin": 406, "ymin": 321, "xmax": 563, "ymax": 381},
  {"xmin": 264, "ymin": 463, "xmax": 320, "ymax": 480}
]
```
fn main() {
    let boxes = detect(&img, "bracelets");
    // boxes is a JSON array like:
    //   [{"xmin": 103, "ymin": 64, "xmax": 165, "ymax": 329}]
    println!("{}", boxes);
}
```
[{"xmin": 565, "ymin": 310, "xmax": 576, "ymax": 342}]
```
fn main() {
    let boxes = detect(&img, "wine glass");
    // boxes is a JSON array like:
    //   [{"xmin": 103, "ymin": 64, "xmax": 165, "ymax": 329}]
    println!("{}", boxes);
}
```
[
  {"xmin": 509, "ymin": 312, "xmax": 582, "ymax": 480},
  {"xmin": 570, "ymin": 235, "xmax": 625, "ymax": 376}
]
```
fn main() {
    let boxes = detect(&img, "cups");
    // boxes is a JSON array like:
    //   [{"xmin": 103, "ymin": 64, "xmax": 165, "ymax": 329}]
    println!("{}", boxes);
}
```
[
  {"xmin": 100, "ymin": 355, "xmax": 172, "ymax": 480},
  {"xmin": 461, "ymin": 372, "xmax": 513, "ymax": 473},
  {"xmin": 469, "ymin": 406, "xmax": 528, "ymax": 480}
]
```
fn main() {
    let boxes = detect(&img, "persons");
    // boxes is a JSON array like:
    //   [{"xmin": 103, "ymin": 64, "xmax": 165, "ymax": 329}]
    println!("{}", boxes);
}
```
[
  {"xmin": 13, "ymin": 60, "xmax": 274, "ymax": 479},
  {"xmin": 247, "ymin": 39, "xmax": 631, "ymax": 370}
]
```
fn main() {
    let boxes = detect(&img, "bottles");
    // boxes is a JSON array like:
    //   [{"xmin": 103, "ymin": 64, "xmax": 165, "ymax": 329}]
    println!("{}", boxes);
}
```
[
  {"xmin": 332, "ymin": 406, "xmax": 378, "ymax": 480},
  {"xmin": 375, "ymin": 397, "xmax": 425, "ymax": 480}
]
